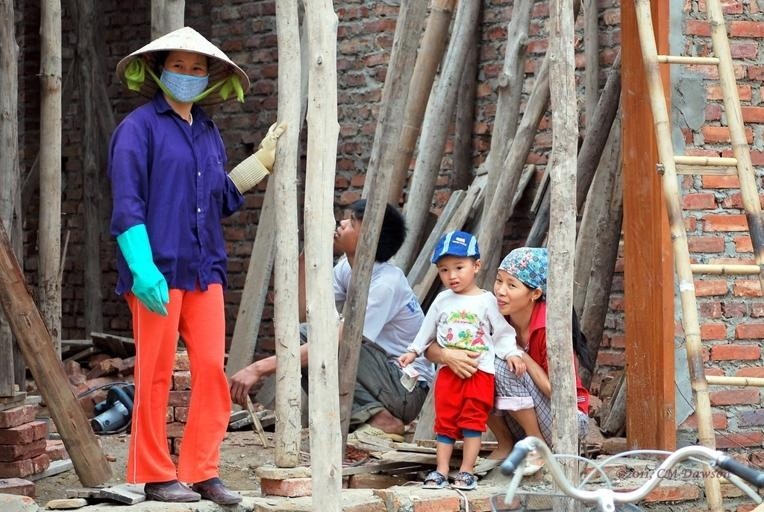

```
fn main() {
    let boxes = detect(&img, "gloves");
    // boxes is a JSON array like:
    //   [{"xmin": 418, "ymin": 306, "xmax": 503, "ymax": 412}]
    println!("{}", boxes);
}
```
[
  {"xmin": 116, "ymin": 223, "xmax": 170, "ymax": 317},
  {"xmin": 227, "ymin": 120, "xmax": 288, "ymax": 196}
]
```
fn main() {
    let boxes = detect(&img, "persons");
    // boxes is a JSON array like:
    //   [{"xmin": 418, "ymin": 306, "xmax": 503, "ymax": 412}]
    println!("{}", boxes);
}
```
[
  {"xmin": 104, "ymin": 25, "xmax": 287, "ymax": 504},
  {"xmin": 397, "ymin": 230, "xmax": 526, "ymax": 490},
  {"xmin": 423, "ymin": 247, "xmax": 590, "ymax": 476},
  {"xmin": 228, "ymin": 198, "xmax": 436, "ymax": 445}
]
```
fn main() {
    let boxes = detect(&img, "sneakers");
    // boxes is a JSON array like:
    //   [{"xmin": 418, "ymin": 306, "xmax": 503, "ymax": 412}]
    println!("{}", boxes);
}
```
[
  {"xmin": 192, "ymin": 477, "xmax": 242, "ymax": 505},
  {"xmin": 144, "ymin": 482, "xmax": 201, "ymax": 502}
]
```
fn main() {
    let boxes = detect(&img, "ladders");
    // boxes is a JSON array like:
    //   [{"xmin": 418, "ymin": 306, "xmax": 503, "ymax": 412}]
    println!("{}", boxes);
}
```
[{"xmin": 631, "ymin": 0, "xmax": 764, "ymax": 512}]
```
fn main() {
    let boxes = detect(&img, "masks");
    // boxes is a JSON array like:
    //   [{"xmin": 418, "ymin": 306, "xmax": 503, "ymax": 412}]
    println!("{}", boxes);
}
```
[{"xmin": 160, "ymin": 69, "xmax": 209, "ymax": 103}]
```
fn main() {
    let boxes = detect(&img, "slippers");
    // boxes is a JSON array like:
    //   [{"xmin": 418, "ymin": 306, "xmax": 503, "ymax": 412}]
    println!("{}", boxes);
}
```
[
  {"xmin": 422, "ymin": 472, "xmax": 449, "ymax": 490},
  {"xmin": 513, "ymin": 460, "xmax": 542, "ymax": 476},
  {"xmin": 346, "ymin": 424, "xmax": 405, "ymax": 445},
  {"xmin": 451, "ymin": 472, "xmax": 477, "ymax": 490},
  {"xmin": 474, "ymin": 457, "xmax": 503, "ymax": 474}
]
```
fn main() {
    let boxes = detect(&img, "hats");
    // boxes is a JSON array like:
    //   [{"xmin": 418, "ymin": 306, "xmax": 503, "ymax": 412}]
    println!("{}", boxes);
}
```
[
  {"xmin": 432, "ymin": 231, "xmax": 480, "ymax": 264},
  {"xmin": 116, "ymin": 26, "xmax": 251, "ymax": 106}
]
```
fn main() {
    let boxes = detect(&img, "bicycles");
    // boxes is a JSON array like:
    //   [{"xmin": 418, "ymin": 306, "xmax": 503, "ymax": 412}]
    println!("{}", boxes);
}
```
[{"xmin": 499, "ymin": 435, "xmax": 764, "ymax": 512}]
